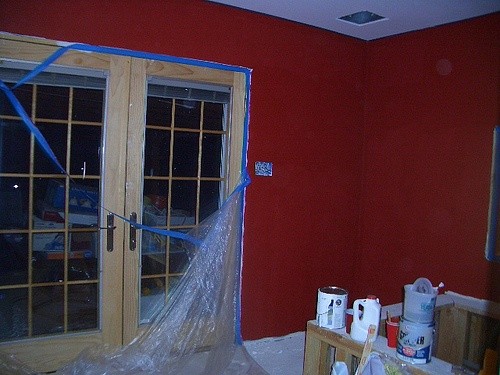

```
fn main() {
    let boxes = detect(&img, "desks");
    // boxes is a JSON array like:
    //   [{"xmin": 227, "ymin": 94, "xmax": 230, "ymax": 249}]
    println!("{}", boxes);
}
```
[{"xmin": 304, "ymin": 295, "xmax": 500, "ymax": 374}]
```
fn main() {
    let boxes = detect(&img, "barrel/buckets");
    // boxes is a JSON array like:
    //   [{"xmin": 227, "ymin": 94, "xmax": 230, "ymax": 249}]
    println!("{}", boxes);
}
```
[
  {"xmin": 404, "ymin": 284, "xmax": 437, "ymax": 324},
  {"xmin": 316, "ymin": 287, "xmax": 348, "ymax": 329},
  {"xmin": 350, "ymin": 295, "xmax": 381, "ymax": 342},
  {"xmin": 396, "ymin": 316, "xmax": 436, "ymax": 364}
]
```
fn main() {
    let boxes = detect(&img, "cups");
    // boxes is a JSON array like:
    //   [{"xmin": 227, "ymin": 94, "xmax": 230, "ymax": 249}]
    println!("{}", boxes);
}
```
[
  {"xmin": 345, "ymin": 308, "xmax": 362, "ymax": 334},
  {"xmin": 385, "ymin": 321, "xmax": 399, "ymax": 348}
]
